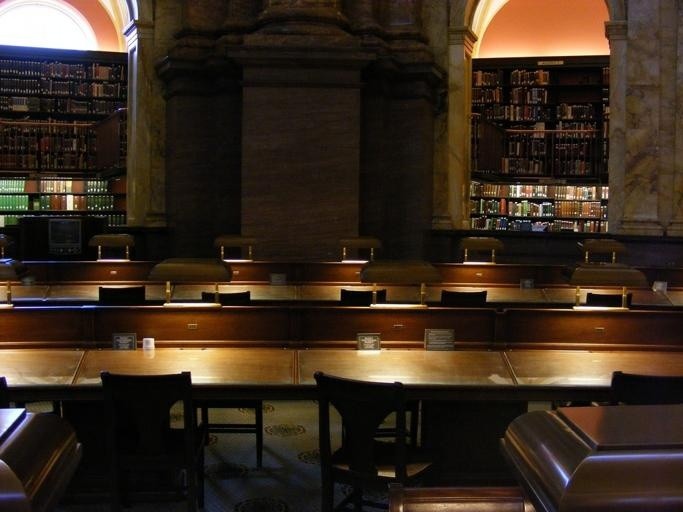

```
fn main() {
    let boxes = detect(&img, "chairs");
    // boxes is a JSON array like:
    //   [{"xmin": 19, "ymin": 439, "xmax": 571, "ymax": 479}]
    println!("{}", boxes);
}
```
[{"xmin": 0, "ymin": 234, "xmax": 683, "ymax": 512}]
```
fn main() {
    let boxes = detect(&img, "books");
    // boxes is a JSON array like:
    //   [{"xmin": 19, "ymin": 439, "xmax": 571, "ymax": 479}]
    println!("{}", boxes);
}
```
[
  {"xmin": 0, "ymin": 119, "xmax": 127, "ymax": 170},
  {"xmin": 1, "ymin": 55, "xmax": 127, "ymax": 116},
  {"xmin": 471, "ymin": 67, "xmax": 551, "ymax": 176},
  {"xmin": 470, "ymin": 181, "xmax": 609, "ymax": 233},
  {"xmin": 0, "ymin": 174, "xmax": 127, "ymax": 227},
  {"xmin": 554, "ymin": 66, "xmax": 610, "ymax": 177}
]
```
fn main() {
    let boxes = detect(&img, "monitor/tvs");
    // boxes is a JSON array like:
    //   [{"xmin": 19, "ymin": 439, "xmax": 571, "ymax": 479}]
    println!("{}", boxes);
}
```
[{"xmin": 48, "ymin": 218, "xmax": 81, "ymax": 256}]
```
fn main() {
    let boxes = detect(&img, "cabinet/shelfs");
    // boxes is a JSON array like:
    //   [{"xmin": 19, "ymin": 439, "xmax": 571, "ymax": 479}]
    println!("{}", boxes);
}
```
[
  {"xmin": 0, "ymin": 45, "xmax": 128, "ymax": 229},
  {"xmin": 469, "ymin": 55, "xmax": 609, "ymax": 233}
]
